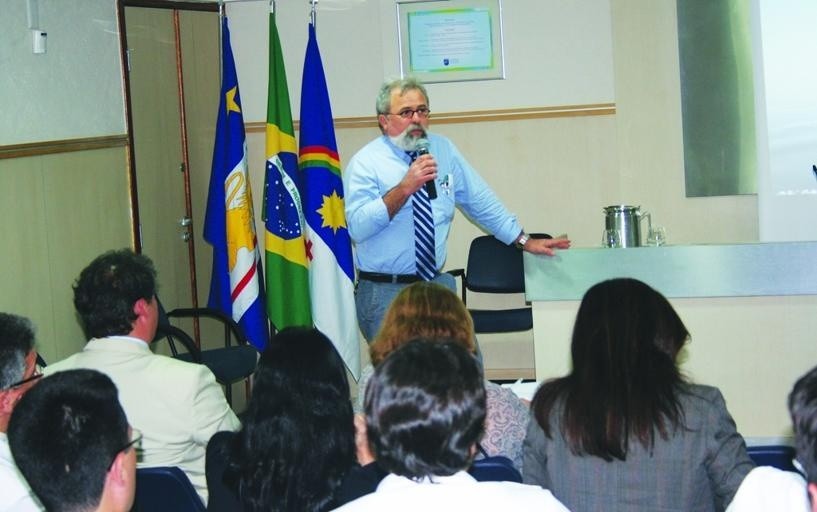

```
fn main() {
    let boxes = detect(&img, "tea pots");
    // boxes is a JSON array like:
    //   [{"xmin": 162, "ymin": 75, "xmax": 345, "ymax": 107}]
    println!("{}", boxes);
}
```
[{"xmin": 603, "ymin": 205, "xmax": 652, "ymax": 248}]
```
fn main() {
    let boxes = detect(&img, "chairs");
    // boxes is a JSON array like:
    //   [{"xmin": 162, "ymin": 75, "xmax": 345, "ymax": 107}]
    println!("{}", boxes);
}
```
[
  {"xmin": 129, "ymin": 439, "xmax": 817, "ymax": 512},
  {"xmin": 150, "ymin": 287, "xmax": 257, "ymax": 407},
  {"xmin": 446, "ymin": 231, "xmax": 554, "ymax": 337}
]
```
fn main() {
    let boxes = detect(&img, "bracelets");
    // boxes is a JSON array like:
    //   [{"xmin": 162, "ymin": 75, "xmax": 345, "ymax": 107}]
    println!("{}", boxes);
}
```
[{"xmin": 516, "ymin": 232, "xmax": 529, "ymax": 247}]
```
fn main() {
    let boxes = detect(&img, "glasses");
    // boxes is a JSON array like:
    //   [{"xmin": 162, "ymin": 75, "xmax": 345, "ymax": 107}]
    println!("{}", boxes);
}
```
[
  {"xmin": 383, "ymin": 104, "xmax": 432, "ymax": 120},
  {"xmin": 4, "ymin": 362, "xmax": 45, "ymax": 389},
  {"xmin": 107, "ymin": 428, "xmax": 144, "ymax": 473}
]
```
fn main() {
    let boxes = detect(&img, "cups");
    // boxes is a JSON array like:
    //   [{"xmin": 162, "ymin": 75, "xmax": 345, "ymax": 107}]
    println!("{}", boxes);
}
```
[
  {"xmin": 600, "ymin": 229, "xmax": 621, "ymax": 248},
  {"xmin": 644, "ymin": 225, "xmax": 666, "ymax": 247}
]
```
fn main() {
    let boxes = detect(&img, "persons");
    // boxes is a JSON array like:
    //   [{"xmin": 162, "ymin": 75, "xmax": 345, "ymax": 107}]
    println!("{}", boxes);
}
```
[
  {"xmin": 1, "ymin": 312, "xmax": 45, "ymax": 510},
  {"xmin": 9, "ymin": 367, "xmax": 140, "ymax": 510},
  {"xmin": 324, "ymin": 336, "xmax": 571, "ymax": 512},
  {"xmin": 202, "ymin": 323, "xmax": 360, "ymax": 510},
  {"xmin": 41, "ymin": 246, "xmax": 240, "ymax": 510},
  {"xmin": 342, "ymin": 77, "xmax": 572, "ymax": 345},
  {"xmin": 520, "ymin": 276, "xmax": 755, "ymax": 511},
  {"xmin": 350, "ymin": 280, "xmax": 532, "ymax": 462},
  {"xmin": 724, "ymin": 363, "xmax": 815, "ymax": 510}
]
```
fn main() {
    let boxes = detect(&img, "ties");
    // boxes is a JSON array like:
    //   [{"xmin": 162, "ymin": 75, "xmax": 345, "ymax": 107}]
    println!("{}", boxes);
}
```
[{"xmin": 406, "ymin": 149, "xmax": 439, "ymax": 281}]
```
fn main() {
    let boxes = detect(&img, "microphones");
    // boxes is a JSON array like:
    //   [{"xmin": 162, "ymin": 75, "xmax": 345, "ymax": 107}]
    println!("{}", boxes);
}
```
[{"xmin": 417, "ymin": 138, "xmax": 440, "ymax": 199}]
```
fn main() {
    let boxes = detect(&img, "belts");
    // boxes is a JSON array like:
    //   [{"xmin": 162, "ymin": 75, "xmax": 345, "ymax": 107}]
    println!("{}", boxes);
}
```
[{"xmin": 358, "ymin": 269, "xmax": 440, "ymax": 285}]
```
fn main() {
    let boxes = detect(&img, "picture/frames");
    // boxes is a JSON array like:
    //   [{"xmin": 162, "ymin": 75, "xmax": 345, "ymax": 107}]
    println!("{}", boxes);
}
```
[{"xmin": 391, "ymin": 1, "xmax": 507, "ymax": 85}]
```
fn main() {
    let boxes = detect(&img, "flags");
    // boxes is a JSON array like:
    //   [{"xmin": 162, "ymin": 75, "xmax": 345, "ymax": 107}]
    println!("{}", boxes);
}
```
[
  {"xmin": 295, "ymin": 8, "xmax": 361, "ymax": 388},
  {"xmin": 200, "ymin": 11, "xmax": 271, "ymax": 355},
  {"xmin": 259, "ymin": 4, "xmax": 313, "ymax": 334}
]
